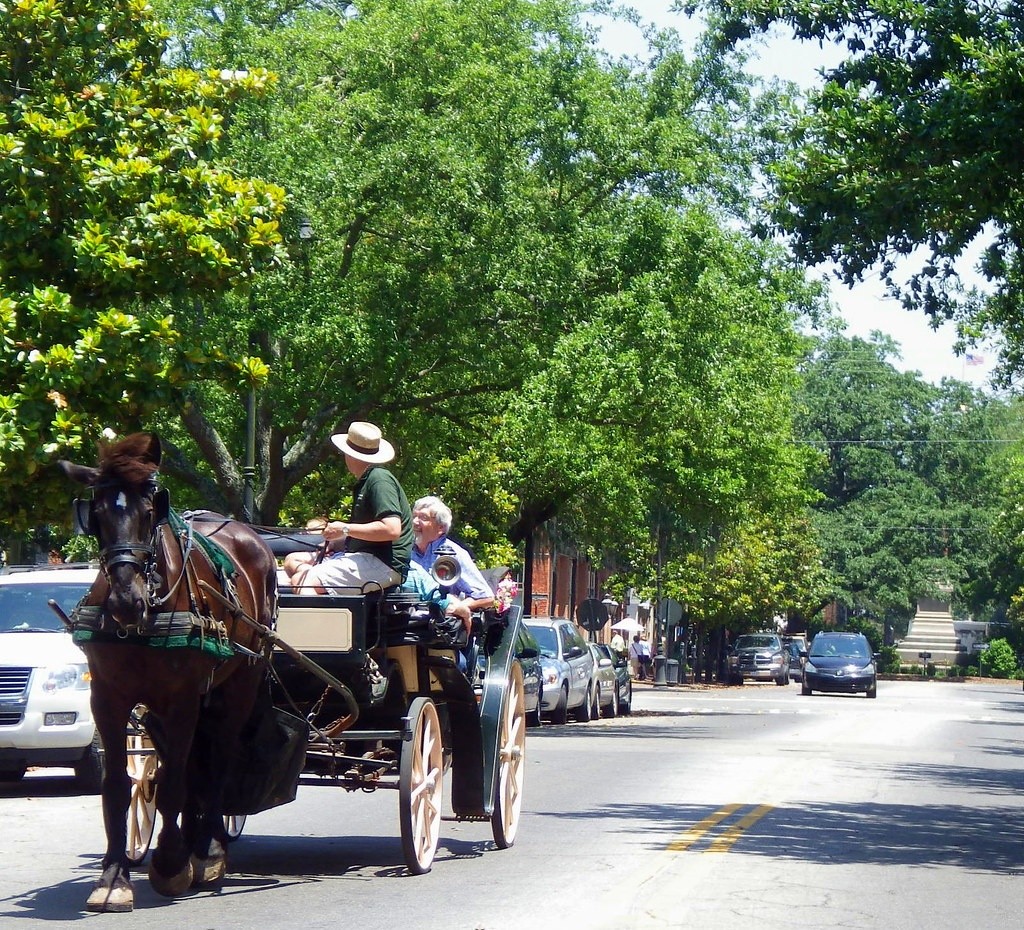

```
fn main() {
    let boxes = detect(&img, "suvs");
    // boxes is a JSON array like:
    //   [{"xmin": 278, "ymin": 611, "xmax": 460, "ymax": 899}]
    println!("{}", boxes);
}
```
[
  {"xmin": 727, "ymin": 632, "xmax": 811, "ymax": 686},
  {"xmin": 0, "ymin": 564, "xmax": 105, "ymax": 794},
  {"xmin": 799, "ymin": 630, "xmax": 881, "ymax": 698}
]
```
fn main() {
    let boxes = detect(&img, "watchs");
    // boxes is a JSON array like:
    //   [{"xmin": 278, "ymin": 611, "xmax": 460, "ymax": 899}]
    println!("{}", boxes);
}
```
[{"xmin": 343, "ymin": 525, "xmax": 350, "ymax": 536}]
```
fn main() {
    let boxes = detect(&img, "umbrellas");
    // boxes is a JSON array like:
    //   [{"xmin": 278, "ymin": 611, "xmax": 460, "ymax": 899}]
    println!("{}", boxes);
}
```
[{"xmin": 610, "ymin": 618, "xmax": 644, "ymax": 644}]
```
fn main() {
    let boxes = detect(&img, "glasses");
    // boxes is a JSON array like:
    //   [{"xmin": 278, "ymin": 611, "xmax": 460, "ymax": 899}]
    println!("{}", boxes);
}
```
[{"xmin": 411, "ymin": 514, "xmax": 437, "ymax": 522}]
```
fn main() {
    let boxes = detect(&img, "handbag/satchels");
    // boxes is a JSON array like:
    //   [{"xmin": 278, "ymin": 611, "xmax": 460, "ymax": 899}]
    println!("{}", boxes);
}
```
[{"xmin": 632, "ymin": 644, "xmax": 649, "ymax": 664}]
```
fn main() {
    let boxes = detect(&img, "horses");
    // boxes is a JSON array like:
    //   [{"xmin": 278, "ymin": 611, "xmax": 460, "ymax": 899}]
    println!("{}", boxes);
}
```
[{"xmin": 59, "ymin": 433, "xmax": 280, "ymax": 913}]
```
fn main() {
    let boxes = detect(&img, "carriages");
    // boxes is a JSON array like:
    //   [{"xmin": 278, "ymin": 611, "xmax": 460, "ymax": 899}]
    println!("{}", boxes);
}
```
[{"xmin": 47, "ymin": 430, "xmax": 526, "ymax": 913}]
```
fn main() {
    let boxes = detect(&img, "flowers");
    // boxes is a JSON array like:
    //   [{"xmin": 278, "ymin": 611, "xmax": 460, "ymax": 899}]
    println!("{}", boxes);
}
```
[{"xmin": 492, "ymin": 572, "xmax": 518, "ymax": 616}]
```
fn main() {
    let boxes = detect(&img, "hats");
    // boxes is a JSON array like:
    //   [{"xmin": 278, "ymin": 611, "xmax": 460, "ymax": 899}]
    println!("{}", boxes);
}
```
[{"xmin": 331, "ymin": 421, "xmax": 395, "ymax": 464}]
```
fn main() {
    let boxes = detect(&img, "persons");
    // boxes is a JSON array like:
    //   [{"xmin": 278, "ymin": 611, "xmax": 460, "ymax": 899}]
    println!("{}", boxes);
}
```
[
  {"xmin": 640, "ymin": 635, "xmax": 651, "ymax": 679},
  {"xmin": 401, "ymin": 560, "xmax": 470, "ymax": 642},
  {"xmin": 611, "ymin": 631, "xmax": 623, "ymax": 656},
  {"xmin": 822, "ymin": 642, "xmax": 835, "ymax": 653},
  {"xmin": 628, "ymin": 636, "xmax": 643, "ymax": 680},
  {"xmin": 307, "ymin": 517, "xmax": 327, "ymax": 534},
  {"xmin": 411, "ymin": 494, "xmax": 495, "ymax": 610},
  {"xmin": 283, "ymin": 422, "xmax": 415, "ymax": 598}
]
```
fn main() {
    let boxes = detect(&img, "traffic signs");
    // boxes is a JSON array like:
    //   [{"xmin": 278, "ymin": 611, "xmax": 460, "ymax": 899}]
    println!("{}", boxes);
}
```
[{"xmin": 973, "ymin": 643, "xmax": 990, "ymax": 650}]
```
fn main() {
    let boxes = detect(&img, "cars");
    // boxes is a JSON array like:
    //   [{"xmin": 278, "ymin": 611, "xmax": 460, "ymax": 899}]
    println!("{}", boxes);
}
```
[{"xmin": 515, "ymin": 614, "xmax": 632, "ymax": 726}]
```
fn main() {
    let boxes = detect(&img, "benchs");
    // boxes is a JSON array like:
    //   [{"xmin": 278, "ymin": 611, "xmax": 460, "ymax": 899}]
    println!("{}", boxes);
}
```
[{"xmin": 258, "ymin": 534, "xmax": 410, "ymax": 594}]
